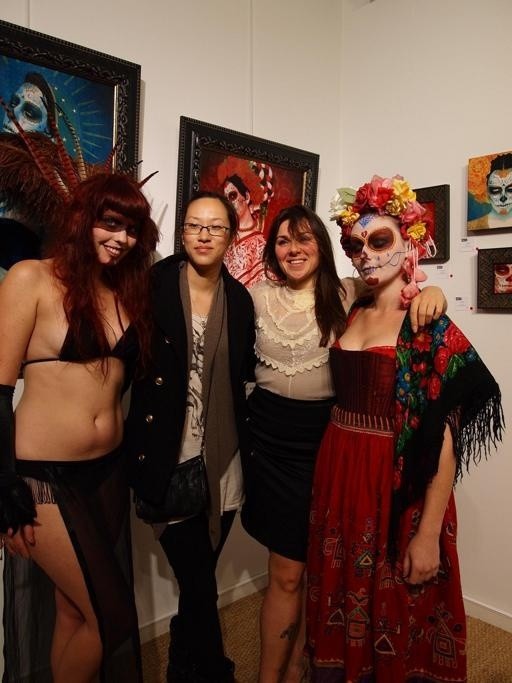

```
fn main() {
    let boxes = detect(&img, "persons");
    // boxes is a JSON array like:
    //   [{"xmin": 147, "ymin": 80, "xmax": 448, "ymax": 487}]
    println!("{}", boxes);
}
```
[
  {"xmin": 2, "ymin": 71, "xmax": 58, "ymax": 136},
  {"xmin": 0, "ymin": 172, "xmax": 161, "ymax": 683},
  {"xmin": 123, "ymin": 193, "xmax": 259, "ymax": 683},
  {"xmin": 200, "ymin": 155, "xmax": 287, "ymax": 288},
  {"xmin": 306, "ymin": 174, "xmax": 506, "ymax": 683},
  {"xmin": 241, "ymin": 205, "xmax": 447, "ymax": 682}
]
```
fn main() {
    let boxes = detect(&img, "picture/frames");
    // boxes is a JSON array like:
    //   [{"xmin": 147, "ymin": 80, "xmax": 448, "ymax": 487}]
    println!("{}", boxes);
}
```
[
  {"xmin": 174, "ymin": 116, "xmax": 320, "ymax": 255},
  {"xmin": 476, "ymin": 246, "xmax": 512, "ymax": 314},
  {"xmin": 412, "ymin": 184, "xmax": 450, "ymax": 264},
  {"xmin": 0, "ymin": 20, "xmax": 142, "ymax": 270}
]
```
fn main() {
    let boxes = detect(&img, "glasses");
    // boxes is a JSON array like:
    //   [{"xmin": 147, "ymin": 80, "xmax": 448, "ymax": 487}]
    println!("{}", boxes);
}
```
[{"xmin": 181, "ymin": 222, "xmax": 231, "ymax": 237}]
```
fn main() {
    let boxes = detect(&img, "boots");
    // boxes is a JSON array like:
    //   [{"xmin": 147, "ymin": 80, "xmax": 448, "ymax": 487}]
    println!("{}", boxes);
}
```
[
  {"xmin": 200, "ymin": 657, "xmax": 240, "ymax": 683},
  {"xmin": 165, "ymin": 614, "xmax": 199, "ymax": 683}
]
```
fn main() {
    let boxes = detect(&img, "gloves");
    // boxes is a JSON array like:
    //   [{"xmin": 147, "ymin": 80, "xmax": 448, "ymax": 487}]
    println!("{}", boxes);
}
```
[{"xmin": 0, "ymin": 383, "xmax": 38, "ymax": 535}]
